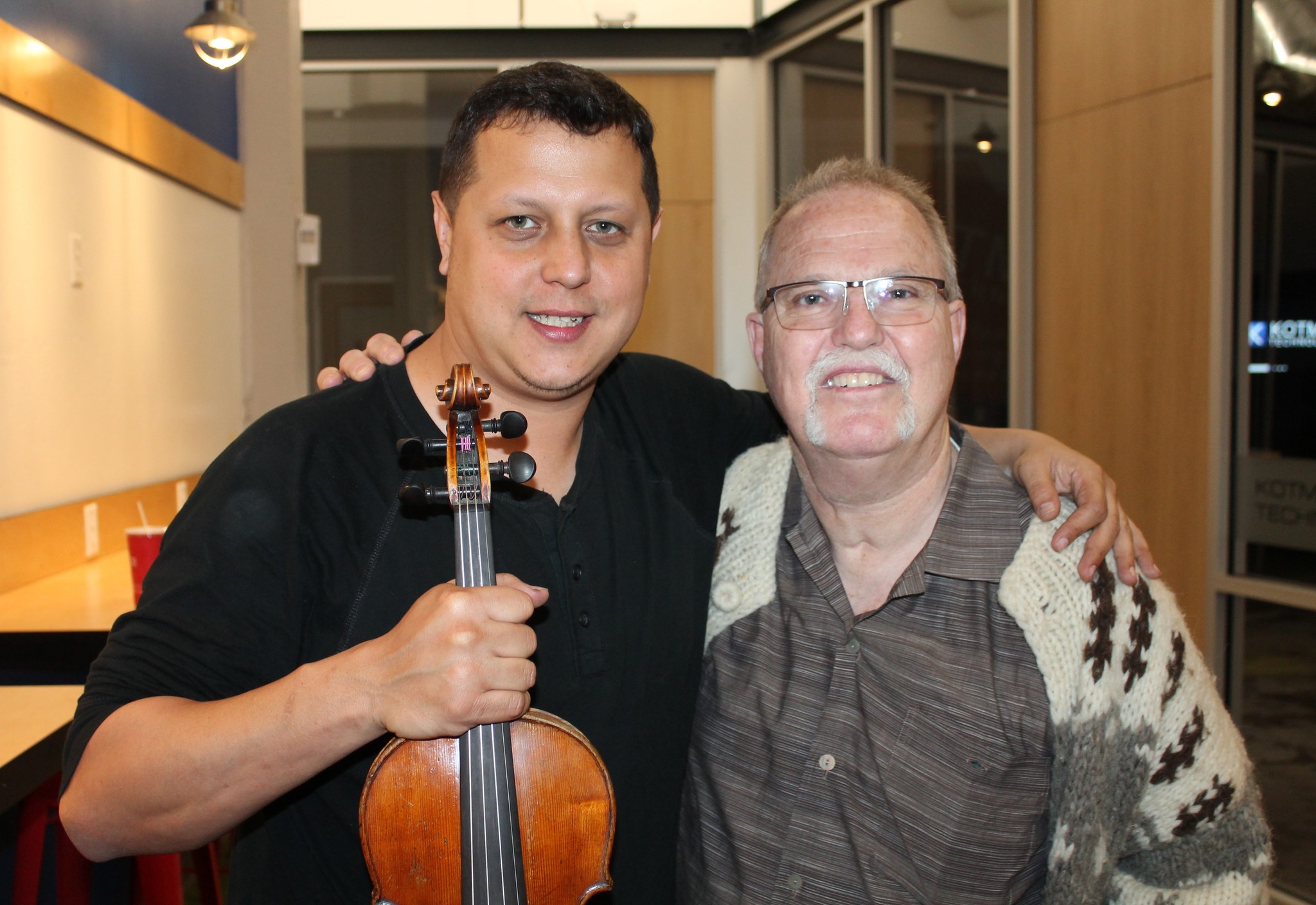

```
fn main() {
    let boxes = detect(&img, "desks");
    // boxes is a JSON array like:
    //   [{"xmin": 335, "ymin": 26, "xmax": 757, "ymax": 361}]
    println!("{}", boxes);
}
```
[
  {"xmin": 0, "ymin": 469, "xmax": 204, "ymax": 634},
  {"xmin": 0, "ymin": 685, "xmax": 86, "ymax": 769}
]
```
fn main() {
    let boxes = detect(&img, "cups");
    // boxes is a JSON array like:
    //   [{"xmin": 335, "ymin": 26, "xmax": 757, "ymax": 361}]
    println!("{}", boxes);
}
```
[{"xmin": 125, "ymin": 527, "xmax": 167, "ymax": 607}]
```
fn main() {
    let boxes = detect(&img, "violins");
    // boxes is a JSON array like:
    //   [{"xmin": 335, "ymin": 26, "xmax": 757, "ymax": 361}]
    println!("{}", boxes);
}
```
[{"xmin": 357, "ymin": 362, "xmax": 618, "ymax": 905}]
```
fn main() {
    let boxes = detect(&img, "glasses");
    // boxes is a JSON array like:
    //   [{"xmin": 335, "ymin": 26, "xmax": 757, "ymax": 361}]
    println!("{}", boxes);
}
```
[{"xmin": 758, "ymin": 275, "xmax": 950, "ymax": 332}]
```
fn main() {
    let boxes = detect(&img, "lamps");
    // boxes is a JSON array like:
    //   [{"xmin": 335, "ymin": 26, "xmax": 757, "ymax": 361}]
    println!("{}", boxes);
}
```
[
  {"xmin": 969, "ymin": 120, "xmax": 1001, "ymax": 155},
  {"xmin": 183, "ymin": 0, "xmax": 257, "ymax": 69}
]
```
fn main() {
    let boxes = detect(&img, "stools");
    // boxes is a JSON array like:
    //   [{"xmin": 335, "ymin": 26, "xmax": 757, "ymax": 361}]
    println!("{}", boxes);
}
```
[{"xmin": 11, "ymin": 773, "xmax": 221, "ymax": 905}]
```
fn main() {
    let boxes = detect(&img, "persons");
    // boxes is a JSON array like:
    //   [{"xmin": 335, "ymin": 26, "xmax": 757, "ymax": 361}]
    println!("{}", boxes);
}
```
[
  {"xmin": 58, "ymin": 61, "xmax": 1163, "ymax": 905},
  {"xmin": 316, "ymin": 157, "xmax": 1272, "ymax": 905}
]
